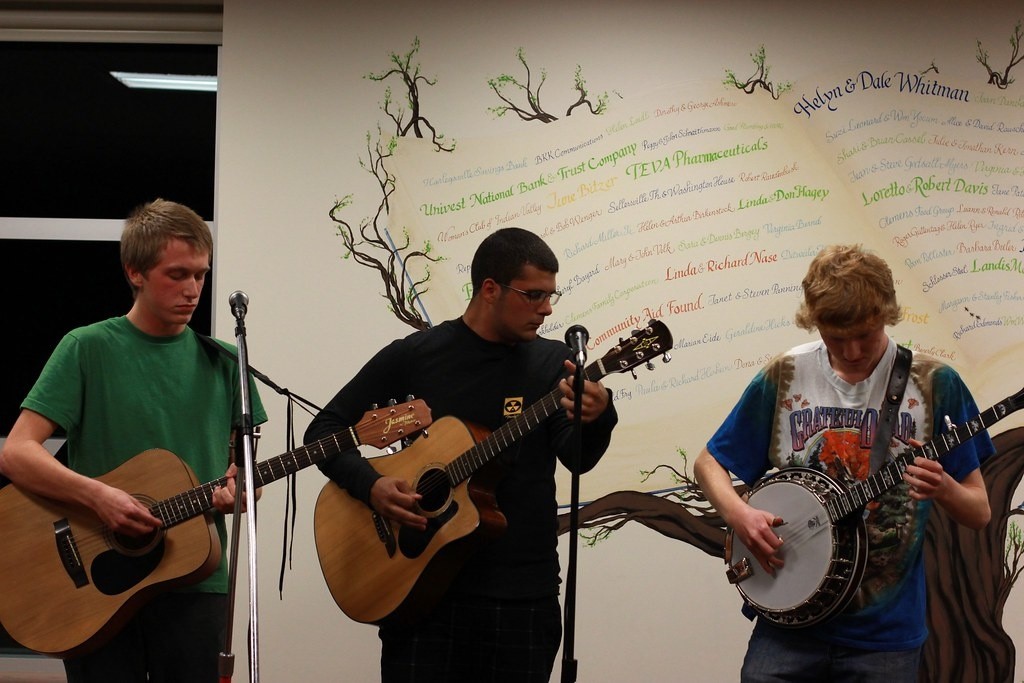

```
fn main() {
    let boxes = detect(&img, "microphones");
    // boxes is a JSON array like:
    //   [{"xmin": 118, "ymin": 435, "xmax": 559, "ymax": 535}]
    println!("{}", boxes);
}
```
[
  {"xmin": 565, "ymin": 324, "xmax": 591, "ymax": 369},
  {"xmin": 229, "ymin": 290, "xmax": 250, "ymax": 320}
]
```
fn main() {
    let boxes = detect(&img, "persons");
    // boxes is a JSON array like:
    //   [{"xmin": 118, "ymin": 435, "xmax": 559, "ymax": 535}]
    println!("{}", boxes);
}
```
[
  {"xmin": 0, "ymin": 198, "xmax": 267, "ymax": 683},
  {"xmin": 304, "ymin": 228, "xmax": 619, "ymax": 683},
  {"xmin": 694, "ymin": 245, "xmax": 996, "ymax": 683}
]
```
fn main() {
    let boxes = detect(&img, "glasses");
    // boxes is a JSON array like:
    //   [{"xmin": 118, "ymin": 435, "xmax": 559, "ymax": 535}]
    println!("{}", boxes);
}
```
[{"xmin": 496, "ymin": 280, "xmax": 562, "ymax": 306}]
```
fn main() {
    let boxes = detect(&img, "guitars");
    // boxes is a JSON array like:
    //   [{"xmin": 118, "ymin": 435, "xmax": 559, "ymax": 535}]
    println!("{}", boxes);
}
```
[
  {"xmin": 723, "ymin": 387, "xmax": 1024, "ymax": 632},
  {"xmin": 312, "ymin": 319, "xmax": 673, "ymax": 624},
  {"xmin": 0, "ymin": 395, "xmax": 434, "ymax": 657}
]
